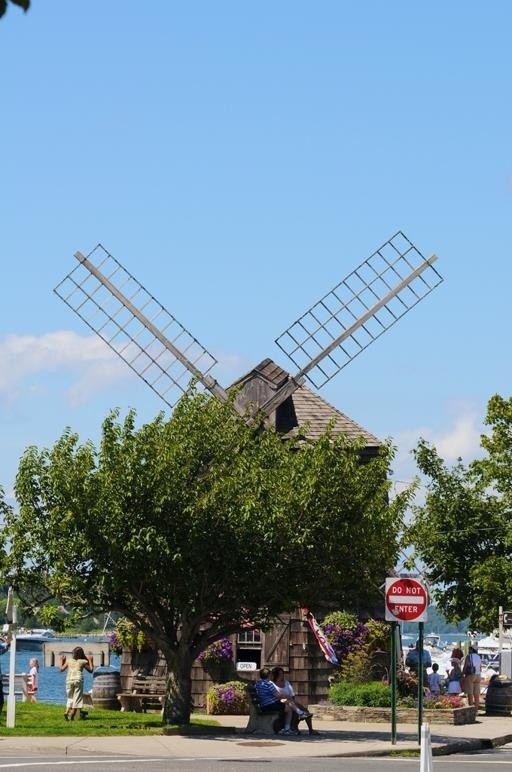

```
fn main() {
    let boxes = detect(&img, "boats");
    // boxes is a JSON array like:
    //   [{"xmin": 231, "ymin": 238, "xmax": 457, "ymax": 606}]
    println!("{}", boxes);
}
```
[{"xmin": 424, "ymin": 633, "xmax": 447, "ymax": 657}]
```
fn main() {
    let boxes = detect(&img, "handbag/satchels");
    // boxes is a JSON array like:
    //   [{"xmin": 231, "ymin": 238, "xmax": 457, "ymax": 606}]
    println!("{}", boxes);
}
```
[{"xmin": 463, "ymin": 654, "xmax": 476, "ymax": 675}]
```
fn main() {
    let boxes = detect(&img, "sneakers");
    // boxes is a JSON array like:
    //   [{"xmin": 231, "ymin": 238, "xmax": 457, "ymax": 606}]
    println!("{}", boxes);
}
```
[
  {"xmin": 63, "ymin": 712, "xmax": 75, "ymax": 722},
  {"xmin": 308, "ymin": 730, "xmax": 324, "ymax": 737},
  {"xmin": 298, "ymin": 712, "xmax": 313, "ymax": 721},
  {"xmin": 281, "ymin": 728, "xmax": 301, "ymax": 737}
]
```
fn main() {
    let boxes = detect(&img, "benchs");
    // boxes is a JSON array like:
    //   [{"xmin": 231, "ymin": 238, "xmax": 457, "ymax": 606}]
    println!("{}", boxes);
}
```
[
  {"xmin": 0, "ymin": 672, "xmax": 35, "ymax": 703},
  {"xmin": 244, "ymin": 686, "xmax": 295, "ymax": 735},
  {"xmin": 115, "ymin": 672, "xmax": 169, "ymax": 716}
]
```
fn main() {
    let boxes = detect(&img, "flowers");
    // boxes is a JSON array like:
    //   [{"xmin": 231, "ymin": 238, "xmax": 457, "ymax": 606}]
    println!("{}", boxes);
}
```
[
  {"xmin": 217, "ymin": 687, "xmax": 239, "ymax": 705},
  {"xmin": 108, "ymin": 633, "xmax": 124, "ymax": 653},
  {"xmin": 195, "ymin": 638, "xmax": 232, "ymax": 664},
  {"xmin": 324, "ymin": 623, "xmax": 370, "ymax": 662}
]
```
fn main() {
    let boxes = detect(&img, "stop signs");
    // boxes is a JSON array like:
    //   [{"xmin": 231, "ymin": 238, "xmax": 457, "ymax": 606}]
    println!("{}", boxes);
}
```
[{"xmin": 384, "ymin": 576, "xmax": 429, "ymax": 622}]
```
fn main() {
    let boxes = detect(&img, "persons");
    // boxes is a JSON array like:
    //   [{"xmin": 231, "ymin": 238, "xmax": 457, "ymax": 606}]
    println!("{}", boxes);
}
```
[
  {"xmin": 271, "ymin": 667, "xmax": 320, "ymax": 735},
  {"xmin": 255, "ymin": 667, "xmax": 313, "ymax": 735},
  {"xmin": 26, "ymin": 657, "xmax": 40, "ymax": 703},
  {"xmin": 461, "ymin": 645, "xmax": 482, "ymax": 715},
  {"xmin": 405, "ymin": 638, "xmax": 431, "ymax": 689},
  {"xmin": 0, "ymin": 637, "xmax": 10, "ymax": 725},
  {"xmin": 60, "ymin": 646, "xmax": 94, "ymax": 720},
  {"xmin": 445, "ymin": 658, "xmax": 462, "ymax": 696},
  {"xmin": 427, "ymin": 663, "xmax": 441, "ymax": 695}
]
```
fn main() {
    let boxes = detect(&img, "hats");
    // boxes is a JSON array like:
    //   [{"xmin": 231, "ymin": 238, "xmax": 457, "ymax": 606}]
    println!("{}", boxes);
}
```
[
  {"xmin": 449, "ymin": 658, "xmax": 462, "ymax": 664},
  {"xmin": 469, "ymin": 645, "xmax": 478, "ymax": 653}
]
